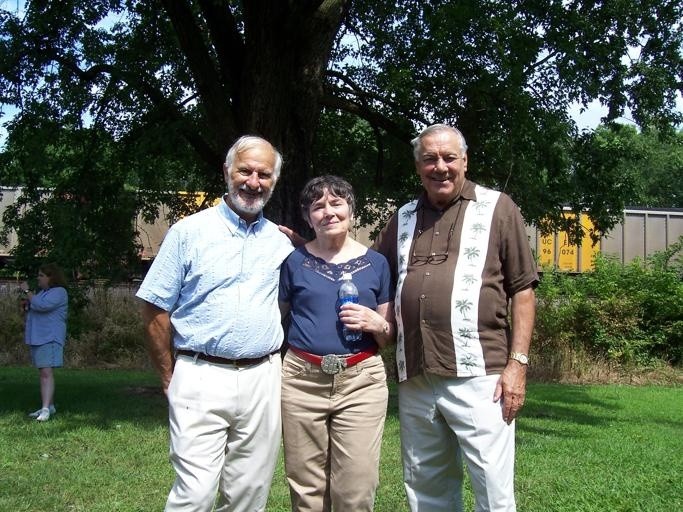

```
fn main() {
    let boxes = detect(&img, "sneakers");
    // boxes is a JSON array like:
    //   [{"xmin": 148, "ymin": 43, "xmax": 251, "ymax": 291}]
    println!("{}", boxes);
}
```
[{"xmin": 29, "ymin": 405, "xmax": 57, "ymax": 422}]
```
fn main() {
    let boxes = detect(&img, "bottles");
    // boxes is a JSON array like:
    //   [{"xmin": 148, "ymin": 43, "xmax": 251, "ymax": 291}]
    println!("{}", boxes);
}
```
[{"xmin": 338, "ymin": 273, "xmax": 363, "ymax": 344}]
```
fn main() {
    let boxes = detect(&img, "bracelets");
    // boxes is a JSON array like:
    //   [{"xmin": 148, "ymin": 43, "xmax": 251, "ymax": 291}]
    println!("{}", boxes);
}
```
[
  {"xmin": 23, "ymin": 289, "xmax": 31, "ymax": 295},
  {"xmin": 380, "ymin": 320, "xmax": 390, "ymax": 339}
]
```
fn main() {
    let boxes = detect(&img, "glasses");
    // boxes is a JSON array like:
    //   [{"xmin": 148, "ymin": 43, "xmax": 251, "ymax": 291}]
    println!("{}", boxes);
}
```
[{"xmin": 411, "ymin": 222, "xmax": 454, "ymax": 262}]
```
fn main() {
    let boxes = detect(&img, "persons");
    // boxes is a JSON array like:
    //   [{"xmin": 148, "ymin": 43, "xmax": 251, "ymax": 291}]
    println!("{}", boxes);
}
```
[
  {"xmin": 277, "ymin": 172, "xmax": 398, "ymax": 510},
  {"xmin": 278, "ymin": 123, "xmax": 542, "ymax": 511},
  {"xmin": 133, "ymin": 133, "xmax": 295, "ymax": 511},
  {"xmin": 16, "ymin": 260, "xmax": 70, "ymax": 423}
]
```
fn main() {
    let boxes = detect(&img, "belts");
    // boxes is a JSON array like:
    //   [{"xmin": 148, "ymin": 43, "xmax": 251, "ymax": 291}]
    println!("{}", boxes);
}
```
[
  {"xmin": 178, "ymin": 349, "xmax": 280, "ymax": 367},
  {"xmin": 286, "ymin": 343, "xmax": 377, "ymax": 376}
]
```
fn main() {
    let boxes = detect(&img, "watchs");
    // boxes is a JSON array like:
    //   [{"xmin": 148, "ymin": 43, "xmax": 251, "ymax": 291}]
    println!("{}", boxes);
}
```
[{"xmin": 508, "ymin": 351, "xmax": 530, "ymax": 366}]
